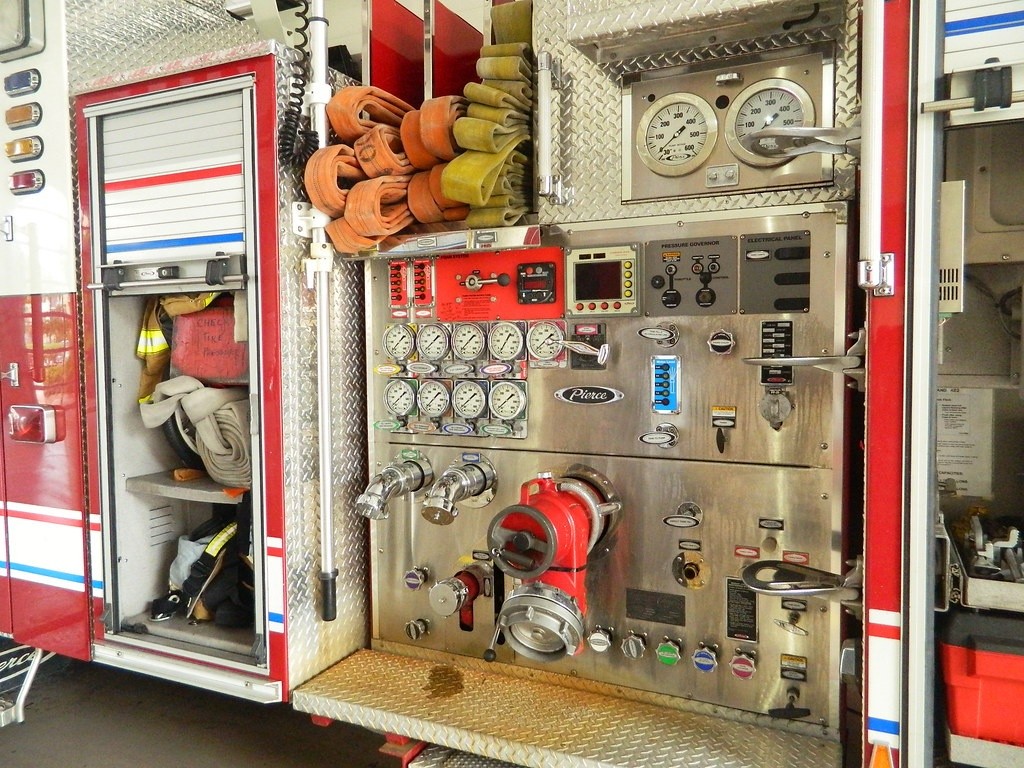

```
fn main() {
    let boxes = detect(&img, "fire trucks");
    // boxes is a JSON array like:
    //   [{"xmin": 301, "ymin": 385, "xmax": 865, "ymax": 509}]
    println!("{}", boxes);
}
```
[{"xmin": 0, "ymin": 0, "xmax": 1024, "ymax": 768}]
